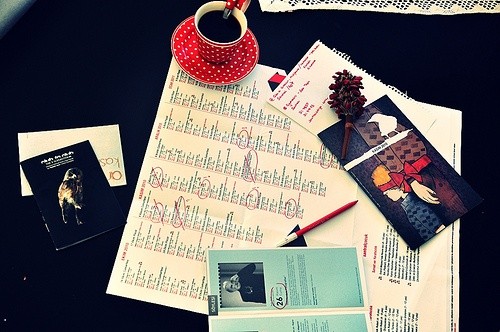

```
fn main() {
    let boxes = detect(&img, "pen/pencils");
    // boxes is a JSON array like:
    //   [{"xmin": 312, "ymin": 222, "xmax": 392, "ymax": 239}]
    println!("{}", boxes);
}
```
[{"xmin": 275, "ymin": 200, "xmax": 360, "ymax": 247}]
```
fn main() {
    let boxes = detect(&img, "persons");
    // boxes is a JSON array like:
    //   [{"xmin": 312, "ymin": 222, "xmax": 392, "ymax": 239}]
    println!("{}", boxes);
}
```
[{"xmin": 222, "ymin": 264, "xmax": 265, "ymax": 305}]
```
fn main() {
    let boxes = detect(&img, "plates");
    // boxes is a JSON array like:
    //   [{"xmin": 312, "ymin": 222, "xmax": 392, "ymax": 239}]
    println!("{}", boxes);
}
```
[{"xmin": 170, "ymin": 15, "xmax": 261, "ymax": 86}]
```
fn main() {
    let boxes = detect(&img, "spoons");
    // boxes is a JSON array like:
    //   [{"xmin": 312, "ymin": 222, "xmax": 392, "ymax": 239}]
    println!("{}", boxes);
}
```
[{"xmin": 223, "ymin": 0, "xmax": 237, "ymax": 20}]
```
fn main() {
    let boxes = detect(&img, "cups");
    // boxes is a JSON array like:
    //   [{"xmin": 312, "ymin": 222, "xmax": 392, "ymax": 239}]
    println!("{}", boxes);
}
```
[{"xmin": 193, "ymin": 0, "xmax": 252, "ymax": 65}]
